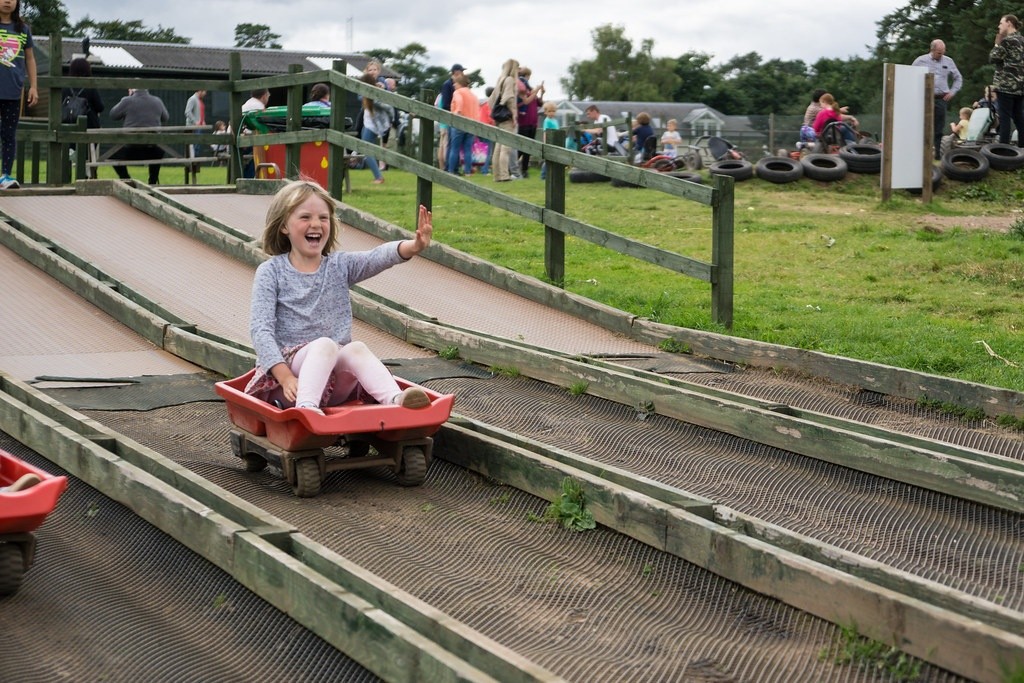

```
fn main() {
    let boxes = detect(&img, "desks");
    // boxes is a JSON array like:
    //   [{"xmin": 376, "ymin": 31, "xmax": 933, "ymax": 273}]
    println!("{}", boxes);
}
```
[{"xmin": 87, "ymin": 126, "xmax": 213, "ymax": 185}]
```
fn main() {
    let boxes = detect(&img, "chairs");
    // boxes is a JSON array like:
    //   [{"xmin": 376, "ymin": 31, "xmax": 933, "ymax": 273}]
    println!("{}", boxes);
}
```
[
  {"xmin": 965, "ymin": 107, "xmax": 993, "ymax": 141},
  {"xmin": 640, "ymin": 136, "xmax": 657, "ymax": 162},
  {"xmin": 824, "ymin": 119, "xmax": 847, "ymax": 147},
  {"xmin": 708, "ymin": 136, "xmax": 733, "ymax": 161}
]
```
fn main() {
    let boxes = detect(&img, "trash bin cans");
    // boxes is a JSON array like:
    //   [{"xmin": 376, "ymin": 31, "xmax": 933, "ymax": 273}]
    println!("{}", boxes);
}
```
[{"xmin": 245, "ymin": 103, "xmax": 354, "ymax": 193}]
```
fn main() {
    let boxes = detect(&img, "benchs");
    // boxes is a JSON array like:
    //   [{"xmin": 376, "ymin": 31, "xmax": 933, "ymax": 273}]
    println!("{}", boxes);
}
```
[{"xmin": 86, "ymin": 156, "xmax": 218, "ymax": 166}]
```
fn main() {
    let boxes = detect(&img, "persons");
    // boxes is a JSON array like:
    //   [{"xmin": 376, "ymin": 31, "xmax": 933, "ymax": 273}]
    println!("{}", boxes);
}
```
[
  {"xmin": 250, "ymin": 178, "xmax": 434, "ymax": 418},
  {"xmin": 0, "ymin": 0, "xmax": 1024, "ymax": 198}
]
